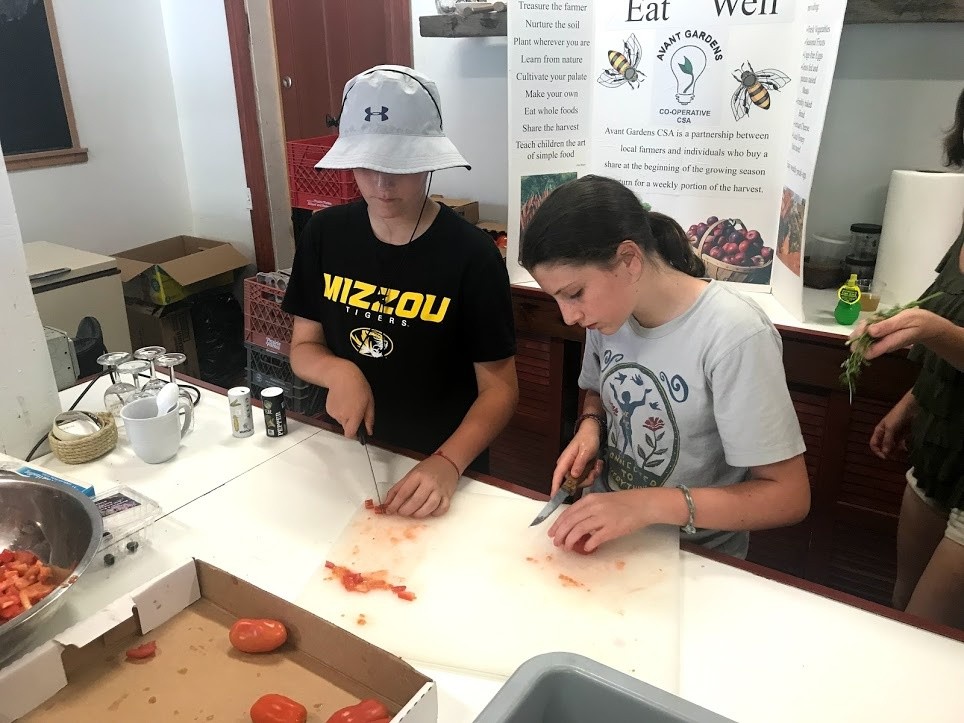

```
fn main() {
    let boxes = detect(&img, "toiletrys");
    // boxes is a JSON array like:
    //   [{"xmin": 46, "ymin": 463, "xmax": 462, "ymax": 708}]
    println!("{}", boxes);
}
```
[{"xmin": 833, "ymin": 273, "xmax": 861, "ymax": 325}]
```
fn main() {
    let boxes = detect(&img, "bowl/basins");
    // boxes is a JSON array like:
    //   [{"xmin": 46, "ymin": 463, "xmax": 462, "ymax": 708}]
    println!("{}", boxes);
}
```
[
  {"xmin": 845, "ymin": 220, "xmax": 881, "ymax": 294},
  {"xmin": 810, "ymin": 232, "xmax": 848, "ymax": 267},
  {"xmin": 802, "ymin": 256, "xmax": 844, "ymax": 290},
  {"xmin": 82, "ymin": 487, "xmax": 161, "ymax": 576},
  {"xmin": 0, "ymin": 476, "xmax": 103, "ymax": 665}
]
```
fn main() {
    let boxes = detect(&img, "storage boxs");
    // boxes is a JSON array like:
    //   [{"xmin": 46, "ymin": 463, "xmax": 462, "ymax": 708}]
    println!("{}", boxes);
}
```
[
  {"xmin": 471, "ymin": 651, "xmax": 740, "ymax": 722},
  {"xmin": 0, "ymin": 559, "xmax": 438, "ymax": 722},
  {"xmin": 106, "ymin": 232, "xmax": 329, "ymax": 420},
  {"xmin": 285, "ymin": 140, "xmax": 480, "ymax": 224}
]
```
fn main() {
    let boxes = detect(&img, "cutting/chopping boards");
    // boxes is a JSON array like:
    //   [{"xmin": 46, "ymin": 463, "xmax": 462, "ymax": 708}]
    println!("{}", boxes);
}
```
[{"xmin": 296, "ymin": 479, "xmax": 682, "ymax": 701}]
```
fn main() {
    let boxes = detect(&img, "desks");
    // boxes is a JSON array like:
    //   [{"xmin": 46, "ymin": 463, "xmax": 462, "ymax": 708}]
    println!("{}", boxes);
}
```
[{"xmin": 0, "ymin": 361, "xmax": 964, "ymax": 723}]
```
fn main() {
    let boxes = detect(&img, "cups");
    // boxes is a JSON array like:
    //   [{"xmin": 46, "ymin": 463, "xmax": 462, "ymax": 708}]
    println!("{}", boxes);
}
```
[
  {"xmin": 119, "ymin": 396, "xmax": 192, "ymax": 463},
  {"xmin": 855, "ymin": 278, "xmax": 887, "ymax": 312}
]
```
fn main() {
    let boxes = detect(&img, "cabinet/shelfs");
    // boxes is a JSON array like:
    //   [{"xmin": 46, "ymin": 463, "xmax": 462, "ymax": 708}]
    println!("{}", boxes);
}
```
[
  {"xmin": 488, "ymin": 285, "xmax": 916, "ymax": 607},
  {"xmin": 25, "ymin": 240, "xmax": 133, "ymax": 380}
]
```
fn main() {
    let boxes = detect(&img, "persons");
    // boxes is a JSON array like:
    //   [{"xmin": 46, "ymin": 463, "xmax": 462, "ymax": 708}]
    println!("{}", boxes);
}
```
[
  {"xmin": 279, "ymin": 65, "xmax": 520, "ymax": 518},
  {"xmin": 517, "ymin": 174, "xmax": 812, "ymax": 563},
  {"xmin": 841, "ymin": 87, "xmax": 964, "ymax": 631}
]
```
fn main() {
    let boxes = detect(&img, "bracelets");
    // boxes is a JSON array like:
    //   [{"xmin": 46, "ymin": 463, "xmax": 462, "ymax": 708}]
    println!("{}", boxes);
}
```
[
  {"xmin": 676, "ymin": 484, "xmax": 696, "ymax": 534},
  {"xmin": 433, "ymin": 450, "xmax": 460, "ymax": 481},
  {"xmin": 573, "ymin": 413, "xmax": 607, "ymax": 451}
]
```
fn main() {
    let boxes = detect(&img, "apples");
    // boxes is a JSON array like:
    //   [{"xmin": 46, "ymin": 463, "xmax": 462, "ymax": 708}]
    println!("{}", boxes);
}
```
[{"xmin": 688, "ymin": 215, "xmax": 774, "ymax": 268}]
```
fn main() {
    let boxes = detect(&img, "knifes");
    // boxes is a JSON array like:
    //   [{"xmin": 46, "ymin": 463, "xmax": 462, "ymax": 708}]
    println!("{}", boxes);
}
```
[
  {"xmin": 529, "ymin": 458, "xmax": 597, "ymax": 527},
  {"xmin": 355, "ymin": 421, "xmax": 384, "ymax": 508}
]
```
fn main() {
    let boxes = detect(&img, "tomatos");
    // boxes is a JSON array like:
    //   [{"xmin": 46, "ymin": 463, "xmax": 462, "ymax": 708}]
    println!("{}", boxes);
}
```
[
  {"xmin": 250, "ymin": 694, "xmax": 307, "ymax": 723},
  {"xmin": 125, "ymin": 642, "xmax": 156, "ymax": 658},
  {"xmin": 228, "ymin": 618, "xmax": 288, "ymax": 653},
  {"xmin": 0, "ymin": 549, "xmax": 55, "ymax": 625},
  {"xmin": 326, "ymin": 698, "xmax": 394, "ymax": 723},
  {"xmin": 365, "ymin": 499, "xmax": 391, "ymax": 514}
]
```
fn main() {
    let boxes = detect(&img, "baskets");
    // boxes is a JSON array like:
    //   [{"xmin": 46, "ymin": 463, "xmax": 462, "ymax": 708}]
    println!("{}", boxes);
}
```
[
  {"xmin": 48, "ymin": 411, "xmax": 118, "ymax": 465},
  {"xmin": 688, "ymin": 219, "xmax": 774, "ymax": 285}
]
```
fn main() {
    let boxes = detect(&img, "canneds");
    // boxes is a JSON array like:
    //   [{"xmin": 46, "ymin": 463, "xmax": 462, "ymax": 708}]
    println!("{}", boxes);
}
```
[
  {"xmin": 261, "ymin": 387, "xmax": 287, "ymax": 437},
  {"xmin": 228, "ymin": 386, "xmax": 255, "ymax": 439}
]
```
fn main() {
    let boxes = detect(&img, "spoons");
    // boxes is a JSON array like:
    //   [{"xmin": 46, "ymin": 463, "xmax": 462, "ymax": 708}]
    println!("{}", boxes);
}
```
[{"xmin": 156, "ymin": 383, "xmax": 179, "ymax": 420}]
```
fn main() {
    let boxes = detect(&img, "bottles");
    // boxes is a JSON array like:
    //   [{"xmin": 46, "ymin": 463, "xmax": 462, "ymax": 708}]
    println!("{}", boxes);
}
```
[{"xmin": 834, "ymin": 274, "xmax": 861, "ymax": 325}]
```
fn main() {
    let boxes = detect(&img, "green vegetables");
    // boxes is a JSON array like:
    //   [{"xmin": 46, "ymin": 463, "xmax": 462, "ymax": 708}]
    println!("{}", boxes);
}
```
[{"xmin": 839, "ymin": 290, "xmax": 946, "ymax": 407}]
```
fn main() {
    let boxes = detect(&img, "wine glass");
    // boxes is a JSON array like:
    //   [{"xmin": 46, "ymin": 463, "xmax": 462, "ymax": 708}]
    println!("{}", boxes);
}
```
[{"xmin": 96, "ymin": 345, "xmax": 195, "ymax": 428}]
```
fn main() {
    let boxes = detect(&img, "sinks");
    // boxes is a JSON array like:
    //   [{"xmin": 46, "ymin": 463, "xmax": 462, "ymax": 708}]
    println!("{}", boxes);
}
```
[{"xmin": 468, "ymin": 652, "xmax": 739, "ymax": 723}]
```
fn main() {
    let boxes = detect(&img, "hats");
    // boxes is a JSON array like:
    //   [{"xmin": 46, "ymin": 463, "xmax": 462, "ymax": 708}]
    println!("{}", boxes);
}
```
[{"xmin": 314, "ymin": 66, "xmax": 472, "ymax": 174}]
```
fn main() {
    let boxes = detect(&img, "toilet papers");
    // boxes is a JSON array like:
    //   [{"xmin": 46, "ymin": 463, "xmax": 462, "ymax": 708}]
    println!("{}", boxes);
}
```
[{"xmin": 869, "ymin": 168, "xmax": 964, "ymax": 318}]
